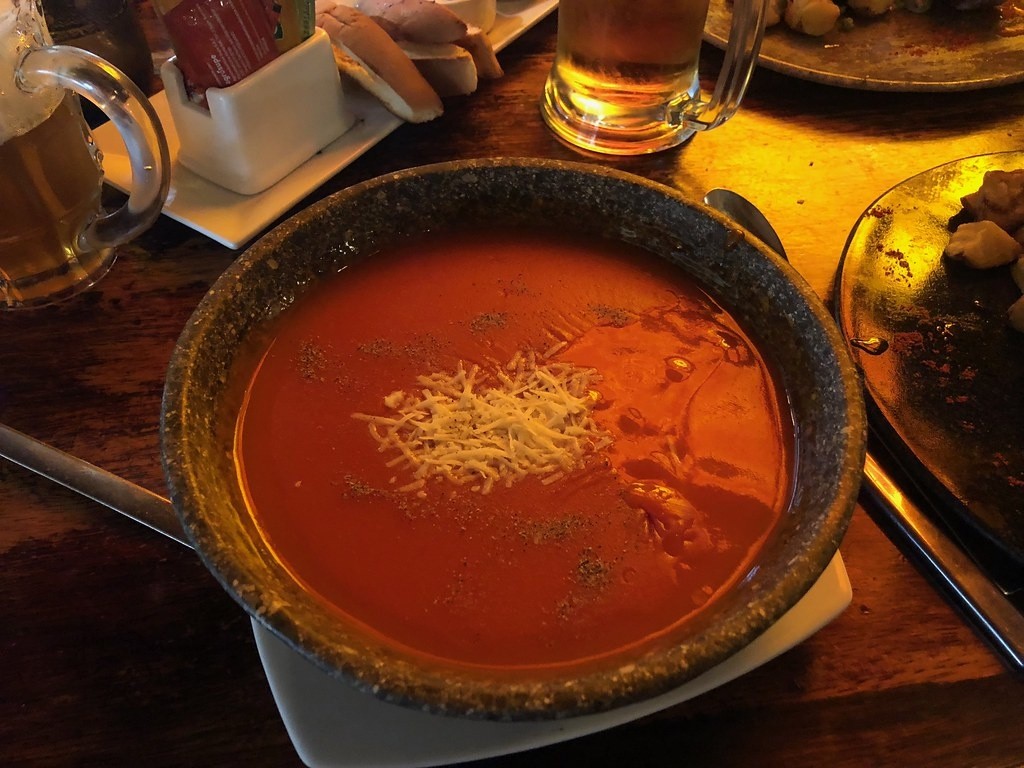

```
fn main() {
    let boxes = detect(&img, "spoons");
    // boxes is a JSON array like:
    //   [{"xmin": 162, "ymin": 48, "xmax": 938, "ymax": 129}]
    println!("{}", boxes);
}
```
[{"xmin": 702, "ymin": 187, "xmax": 1017, "ymax": 600}]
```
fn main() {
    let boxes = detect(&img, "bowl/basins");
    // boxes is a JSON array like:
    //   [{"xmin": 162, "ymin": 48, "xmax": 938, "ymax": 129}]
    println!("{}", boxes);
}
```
[{"xmin": 159, "ymin": 155, "xmax": 867, "ymax": 719}]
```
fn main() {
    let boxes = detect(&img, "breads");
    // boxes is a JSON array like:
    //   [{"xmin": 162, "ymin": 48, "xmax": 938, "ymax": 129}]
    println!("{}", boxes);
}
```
[{"xmin": 314, "ymin": 1, "xmax": 504, "ymax": 124}]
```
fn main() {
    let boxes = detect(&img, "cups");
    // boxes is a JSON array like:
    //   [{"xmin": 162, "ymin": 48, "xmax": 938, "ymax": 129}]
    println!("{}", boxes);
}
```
[
  {"xmin": 540, "ymin": 0, "xmax": 767, "ymax": 156},
  {"xmin": 0, "ymin": 0, "xmax": 171, "ymax": 317}
]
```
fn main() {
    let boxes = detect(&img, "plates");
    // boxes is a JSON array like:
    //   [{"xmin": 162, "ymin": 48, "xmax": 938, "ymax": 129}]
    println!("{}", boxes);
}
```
[
  {"xmin": 90, "ymin": 0, "xmax": 559, "ymax": 251},
  {"xmin": 834, "ymin": 151, "xmax": 1024, "ymax": 567},
  {"xmin": 249, "ymin": 547, "xmax": 855, "ymax": 767},
  {"xmin": 702, "ymin": 0, "xmax": 1024, "ymax": 93}
]
